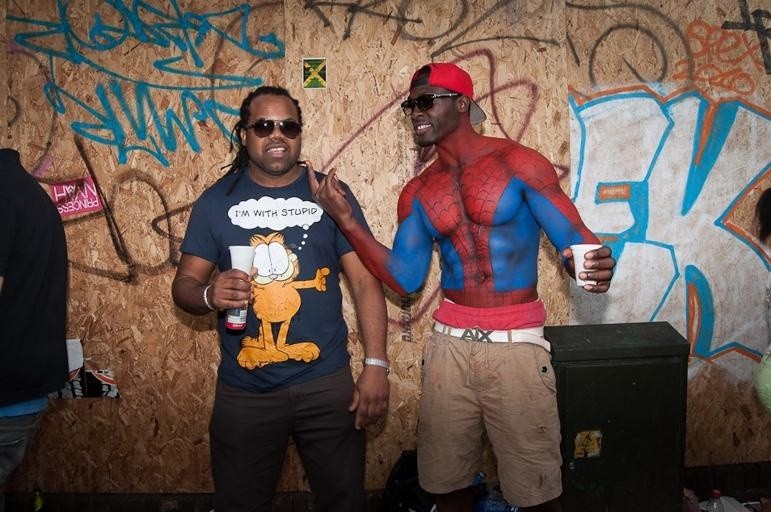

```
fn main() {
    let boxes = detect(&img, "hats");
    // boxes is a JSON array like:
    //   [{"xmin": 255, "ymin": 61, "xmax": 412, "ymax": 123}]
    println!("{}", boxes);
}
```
[{"xmin": 409, "ymin": 63, "xmax": 487, "ymax": 125}]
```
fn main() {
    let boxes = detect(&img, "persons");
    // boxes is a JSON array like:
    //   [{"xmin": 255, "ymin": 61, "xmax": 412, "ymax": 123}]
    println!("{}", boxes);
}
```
[
  {"xmin": 171, "ymin": 85, "xmax": 395, "ymax": 512},
  {"xmin": 304, "ymin": 59, "xmax": 617, "ymax": 511},
  {"xmin": 753, "ymin": 186, "xmax": 771, "ymax": 422},
  {"xmin": 0, "ymin": 146, "xmax": 71, "ymax": 488}
]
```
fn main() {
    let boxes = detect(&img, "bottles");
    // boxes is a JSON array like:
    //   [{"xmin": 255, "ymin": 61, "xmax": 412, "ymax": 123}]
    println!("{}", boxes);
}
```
[{"xmin": 223, "ymin": 277, "xmax": 248, "ymax": 332}]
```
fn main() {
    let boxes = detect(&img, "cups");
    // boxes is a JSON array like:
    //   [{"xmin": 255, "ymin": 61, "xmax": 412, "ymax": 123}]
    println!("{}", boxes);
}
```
[
  {"xmin": 568, "ymin": 243, "xmax": 604, "ymax": 287},
  {"xmin": 228, "ymin": 244, "xmax": 256, "ymax": 277}
]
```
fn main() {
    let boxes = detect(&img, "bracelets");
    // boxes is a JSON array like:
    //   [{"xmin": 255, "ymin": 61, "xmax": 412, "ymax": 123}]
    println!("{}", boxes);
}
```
[
  {"xmin": 363, "ymin": 356, "xmax": 392, "ymax": 376},
  {"xmin": 202, "ymin": 284, "xmax": 217, "ymax": 312}
]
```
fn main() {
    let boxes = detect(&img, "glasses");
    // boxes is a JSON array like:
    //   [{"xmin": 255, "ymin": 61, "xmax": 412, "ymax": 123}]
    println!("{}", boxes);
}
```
[
  {"xmin": 246, "ymin": 119, "xmax": 302, "ymax": 139},
  {"xmin": 400, "ymin": 92, "xmax": 459, "ymax": 116}
]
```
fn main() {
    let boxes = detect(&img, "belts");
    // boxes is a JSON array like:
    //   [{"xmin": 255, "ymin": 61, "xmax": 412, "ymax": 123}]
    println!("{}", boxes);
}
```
[{"xmin": 432, "ymin": 322, "xmax": 552, "ymax": 355}]
